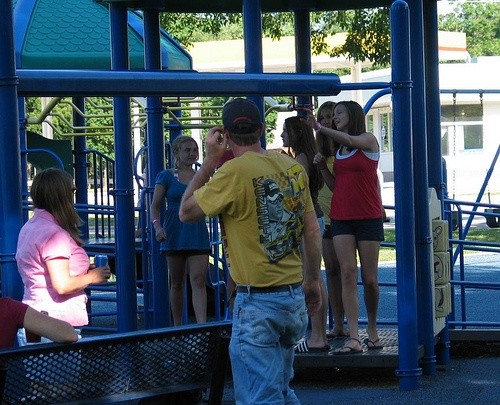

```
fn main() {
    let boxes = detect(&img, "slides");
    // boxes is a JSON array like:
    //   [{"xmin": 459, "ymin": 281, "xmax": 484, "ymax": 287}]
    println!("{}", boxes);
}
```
[{"xmin": 107, "ymin": 249, "xmax": 224, "ymax": 305}]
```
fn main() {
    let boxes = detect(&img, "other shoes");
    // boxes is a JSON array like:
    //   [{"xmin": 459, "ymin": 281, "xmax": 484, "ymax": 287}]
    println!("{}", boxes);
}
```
[{"xmin": 327, "ymin": 332, "xmax": 351, "ymax": 338}]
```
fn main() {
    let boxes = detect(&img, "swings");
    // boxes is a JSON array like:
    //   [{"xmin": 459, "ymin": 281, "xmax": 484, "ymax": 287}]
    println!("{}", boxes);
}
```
[
  {"xmin": 479, "ymin": 92, "xmax": 500, "ymax": 228},
  {"xmin": 452, "ymin": 93, "xmax": 460, "ymax": 230}
]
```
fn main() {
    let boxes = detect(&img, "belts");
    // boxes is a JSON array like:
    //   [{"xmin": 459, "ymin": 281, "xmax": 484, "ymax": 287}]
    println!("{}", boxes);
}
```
[{"xmin": 237, "ymin": 281, "xmax": 302, "ymax": 293}]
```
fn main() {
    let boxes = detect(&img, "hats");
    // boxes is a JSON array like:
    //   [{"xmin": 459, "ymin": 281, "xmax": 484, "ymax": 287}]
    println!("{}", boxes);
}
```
[{"xmin": 222, "ymin": 98, "xmax": 261, "ymax": 134}]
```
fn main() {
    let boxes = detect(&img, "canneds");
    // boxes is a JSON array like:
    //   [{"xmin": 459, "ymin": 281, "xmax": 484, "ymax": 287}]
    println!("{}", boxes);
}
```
[{"xmin": 94, "ymin": 254, "xmax": 108, "ymax": 268}]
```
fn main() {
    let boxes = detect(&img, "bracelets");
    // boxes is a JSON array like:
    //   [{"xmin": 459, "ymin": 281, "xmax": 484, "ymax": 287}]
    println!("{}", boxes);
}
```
[
  {"xmin": 315, "ymin": 121, "xmax": 322, "ymax": 133},
  {"xmin": 152, "ymin": 219, "xmax": 159, "ymax": 225}
]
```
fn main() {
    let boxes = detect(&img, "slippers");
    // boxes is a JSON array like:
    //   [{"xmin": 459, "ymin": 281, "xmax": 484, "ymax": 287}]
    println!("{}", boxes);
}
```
[
  {"xmin": 363, "ymin": 337, "xmax": 384, "ymax": 349},
  {"xmin": 295, "ymin": 339, "xmax": 331, "ymax": 354},
  {"xmin": 333, "ymin": 338, "xmax": 363, "ymax": 355}
]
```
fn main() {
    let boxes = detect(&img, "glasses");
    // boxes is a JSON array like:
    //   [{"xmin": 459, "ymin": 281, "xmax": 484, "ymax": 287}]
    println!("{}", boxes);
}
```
[{"xmin": 71, "ymin": 186, "xmax": 78, "ymax": 195}]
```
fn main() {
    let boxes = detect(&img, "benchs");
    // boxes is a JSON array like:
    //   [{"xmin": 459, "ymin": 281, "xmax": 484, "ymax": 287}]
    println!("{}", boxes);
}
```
[{"xmin": 0, "ymin": 318, "xmax": 234, "ymax": 405}]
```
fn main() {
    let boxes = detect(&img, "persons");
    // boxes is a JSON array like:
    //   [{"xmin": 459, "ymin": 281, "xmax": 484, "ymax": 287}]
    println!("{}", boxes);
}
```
[
  {"xmin": 147, "ymin": 136, "xmax": 210, "ymax": 327},
  {"xmin": 277, "ymin": 101, "xmax": 385, "ymax": 353},
  {"xmin": 0, "ymin": 294, "xmax": 78, "ymax": 405},
  {"xmin": 178, "ymin": 99, "xmax": 323, "ymax": 405},
  {"xmin": 15, "ymin": 168, "xmax": 110, "ymax": 403}
]
